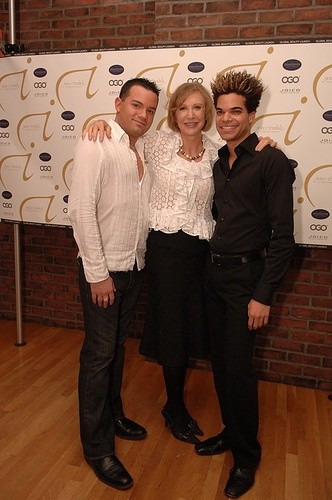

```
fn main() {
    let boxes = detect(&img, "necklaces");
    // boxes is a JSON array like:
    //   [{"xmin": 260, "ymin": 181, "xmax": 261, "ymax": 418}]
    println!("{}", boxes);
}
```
[{"xmin": 179, "ymin": 147, "xmax": 205, "ymax": 160}]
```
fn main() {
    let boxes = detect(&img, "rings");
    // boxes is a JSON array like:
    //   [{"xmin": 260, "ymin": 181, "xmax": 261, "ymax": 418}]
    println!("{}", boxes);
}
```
[{"xmin": 103, "ymin": 297, "xmax": 108, "ymax": 302}]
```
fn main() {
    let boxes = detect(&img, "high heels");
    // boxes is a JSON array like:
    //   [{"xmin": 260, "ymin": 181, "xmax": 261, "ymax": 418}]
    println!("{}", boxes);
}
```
[{"xmin": 162, "ymin": 403, "xmax": 204, "ymax": 445}]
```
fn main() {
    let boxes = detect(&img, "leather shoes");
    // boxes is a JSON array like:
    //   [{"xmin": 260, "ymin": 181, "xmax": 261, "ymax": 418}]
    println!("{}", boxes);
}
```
[
  {"xmin": 224, "ymin": 466, "xmax": 255, "ymax": 497},
  {"xmin": 85, "ymin": 453, "xmax": 133, "ymax": 491},
  {"xmin": 115, "ymin": 418, "xmax": 147, "ymax": 441},
  {"xmin": 194, "ymin": 433, "xmax": 229, "ymax": 456}
]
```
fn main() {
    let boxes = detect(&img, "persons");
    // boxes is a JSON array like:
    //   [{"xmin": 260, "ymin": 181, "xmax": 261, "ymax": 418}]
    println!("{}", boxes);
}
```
[
  {"xmin": 67, "ymin": 78, "xmax": 161, "ymax": 491},
  {"xmin": 81, "ymin": 70, "xmax": 295, "ymax": 498}
]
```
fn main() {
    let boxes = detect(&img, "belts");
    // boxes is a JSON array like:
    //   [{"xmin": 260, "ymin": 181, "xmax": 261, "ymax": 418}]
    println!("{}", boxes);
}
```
[{"xmin": 209, "ymin": 251, "xmax": 266, "ymax": 265}]
